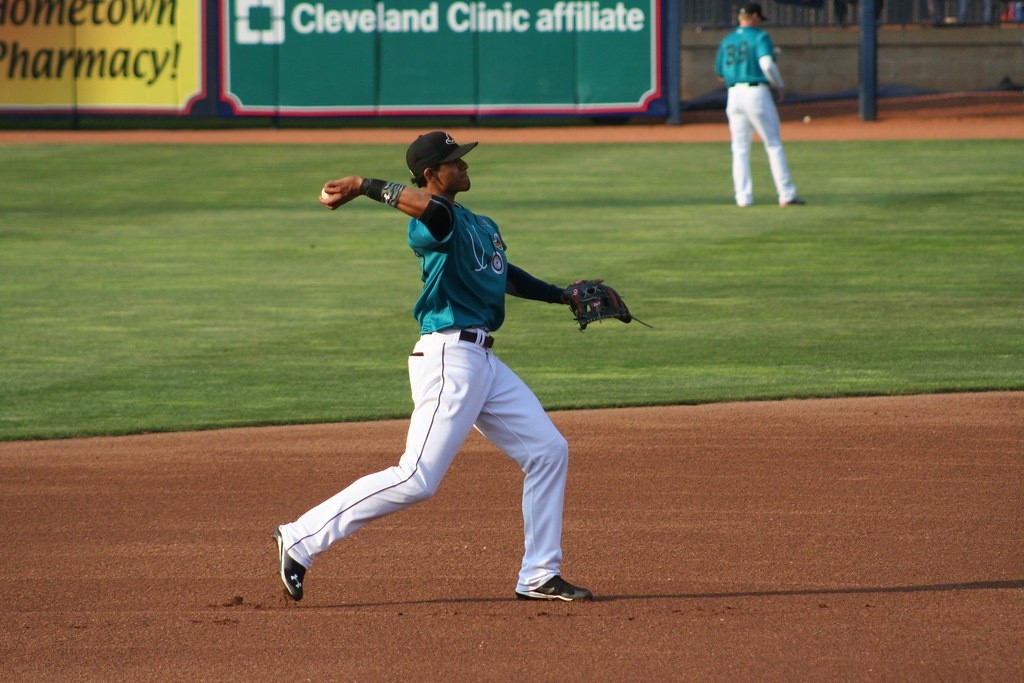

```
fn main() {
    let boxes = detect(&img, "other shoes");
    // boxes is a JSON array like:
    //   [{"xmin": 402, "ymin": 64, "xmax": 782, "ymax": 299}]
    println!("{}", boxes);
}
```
[{"xmin": 781, "ymin": 200, "xmax": 806, "ymax": 206}]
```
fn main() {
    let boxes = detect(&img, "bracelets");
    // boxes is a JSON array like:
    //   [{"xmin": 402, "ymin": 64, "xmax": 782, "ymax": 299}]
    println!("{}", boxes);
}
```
[{"xmin": 359, "ymin": 178, "xmax": 406, "ymax": 208}]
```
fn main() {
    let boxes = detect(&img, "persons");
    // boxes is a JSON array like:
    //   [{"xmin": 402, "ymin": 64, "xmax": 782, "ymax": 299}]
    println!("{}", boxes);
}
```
[
  {"xmin": 269, "ymin": 130, "xmax": 598, "ymax": 605},
  {"xmin": 714, "ymin": 3, "xmax": 806, "ymax": 206}
]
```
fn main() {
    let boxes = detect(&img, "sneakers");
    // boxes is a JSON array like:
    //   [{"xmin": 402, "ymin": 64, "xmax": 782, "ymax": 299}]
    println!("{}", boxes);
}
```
[
  {"xmin": 515, "ymin": 576, "xmax": 592, "ymax": 602},
  {"xmin": 272, "ymin": 526, "xmax": 306, "ymax": 602}
]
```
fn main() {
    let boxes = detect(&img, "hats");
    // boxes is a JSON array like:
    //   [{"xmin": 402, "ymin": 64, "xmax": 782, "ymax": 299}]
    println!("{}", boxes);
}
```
[
  {"xmin": 739, "ymin": 3, "xmax": 768, "ymax": 22},
  {"xmin": 406, "ymin": 132, "xmax": 479, "ymax": 179}
]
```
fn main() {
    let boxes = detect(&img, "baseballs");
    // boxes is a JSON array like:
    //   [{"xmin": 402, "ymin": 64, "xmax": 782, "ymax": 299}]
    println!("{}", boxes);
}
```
[
  {"xmin": 802, "ymin": 114, "xmax": 812, "ymax": 124},
  {"xmin": 320, "ymin": 187, "xmax": 334, "ymax": 200}
]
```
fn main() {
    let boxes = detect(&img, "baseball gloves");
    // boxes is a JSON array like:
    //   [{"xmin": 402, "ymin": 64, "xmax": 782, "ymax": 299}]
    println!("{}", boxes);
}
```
[{"xmin": 563, "ymin": 277, "xmax": 654, "ymax": 334}]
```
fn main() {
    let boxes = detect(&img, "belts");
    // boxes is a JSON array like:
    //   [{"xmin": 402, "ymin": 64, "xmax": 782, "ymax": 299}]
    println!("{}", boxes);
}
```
[
  {"xmin": 731, "ymin": 82, "xmax": 758, "ymax": 87},
  {"xmin": 460, "ymin": 329, "xmax": 495, "ymax": 348}
]
